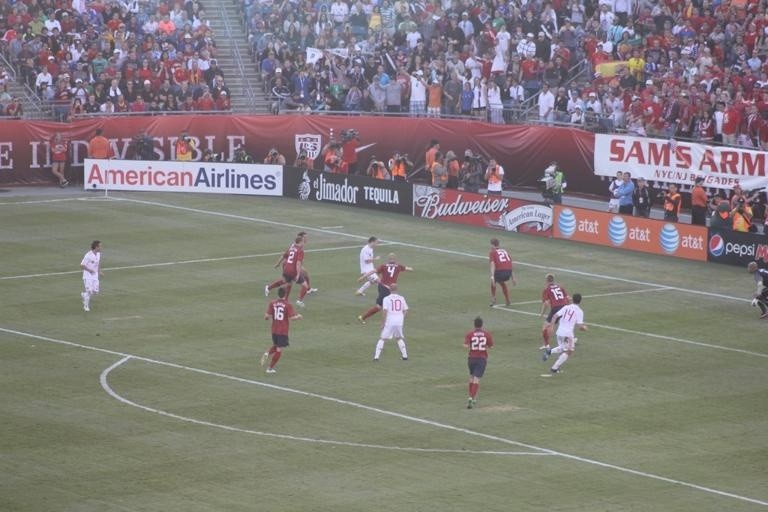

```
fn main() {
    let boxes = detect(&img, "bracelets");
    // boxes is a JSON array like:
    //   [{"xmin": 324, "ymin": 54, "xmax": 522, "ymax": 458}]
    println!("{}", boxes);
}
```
[{"xmin": 491, "ymin": 276, "xmax": 494, "ymax": 280}]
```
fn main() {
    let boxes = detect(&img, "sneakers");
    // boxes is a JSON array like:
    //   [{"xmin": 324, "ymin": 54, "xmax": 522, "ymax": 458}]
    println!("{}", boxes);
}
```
[
  {"xmin": 260, "ymin": 352, "xmax": 275, "ymax": 374},
  {"xmin": 761, "ymin": 311, "xmax": 768, "ymax": 318},
  {"xmin": 400, "ymin": 357, "xmax": 408, "ymax": 360},
  {"xmin": 307, "ymin": 287, "xmax": 318, "ymax": 294},
  {"xmin": 60, "ymin": 181, "xmax": 69, "ymax": 187},
  {"xmin": 373, "ymin": 358, "xmax": 378, "ymax": 361},
  {"xmin": 490, "ymin": 300, "xmax": 495, "ymax": 306},
  {"xmin": 549, "ymin": 367, "xmax": 563, "ymax": 373},
  {"xmin": 468, "ymin": 398, "xmax": 477, "ymax": 410},
  {"xmin": 296, "ymin": 299, "xmax": 304, "ymax": 306},
  {"xmin": 265, "ymin": 284, "xmax": 269, "ymax": 297},
  {"xmin": 539, "ymin": 344, "xmax": 551, "ymax": 360},
  {"xmin": 80, "ymin": 292, "xmax": 91, "ymax": 311},
  {"xmin": 356, "ymin": 291, "xmax": 365, "ymax": 296},
  {"xmin": 357, "ymin": 315, "xmax": 365, "ymax": 325}
]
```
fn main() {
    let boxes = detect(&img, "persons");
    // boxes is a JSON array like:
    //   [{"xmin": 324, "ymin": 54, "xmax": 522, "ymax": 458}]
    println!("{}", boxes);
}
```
[
  {"xmin": 88, "ymin": 128, "xmax": 361, "ymax": 175},
  {"xmin": 462, "ymin": 318, "xmax": 495, "ymax": 409},
  {"xmin": 357, "ymin": 252, "xmax": 414, "ymax": 325},
  {"xmin": 487, "ymin": 238, "xmax": 516, "ymax": 307},
  {"xmin": 426, "ymin": 139, "xmax": 505, "ymax": 194},
  {"xmin": 52, "ymin": 133, "xmax": 69, "ymax": 188},
  {"xmin": 260, "ymin": 287, "xmax": 303, "ymax": 374},
  {"xmin": 543, "ymin": 294, "xmax": 589, "ymax": 373},
  {"xmin": 609, "ymin": 171, "xmax": 652, "ymax": 218},
  {"xmin": 264, "ymin": 232, "xmax": 319, "ymax": 297},
  {"xmin": 662, "ymin": 183, "xmax": 681, "ymax": 223},
  {"xmin": 354, "ymin": 236, "xmax": 381, "ymax": 296},
  {"xmin": 746, "ymin": 261, "xmax": 768, "ymax": 319},
  {"xmin": 538, "ymin": 273, "xmax": 578, "ymax": 350},
  {"xmin": 367, "ymin": 151, "xmax": 414, "ymax": 182},
  {"xmin": 373, "ymin": 282, "xmax": 412, "ymax": 360},
  {"xmin": 272, "ymin": 238, "xmax": 310, "ymax": 307},
  {"xmin": 692, "ymin": 176, "xmax": 753, "ymax": 233},
  {"xmin": 548, "ymin": 160, "xmax": 566, "ymax": 204},
  {"xmin": 79, "ymin": 240, "xmax": 104, "ymax": 311},
  {"xmin": 238, "ymin": 0, "xmax": 767, "ymax": 149},
  {"xmin": 0, "ymin": 0, "xmax": 231, "ymax": 120}
]
